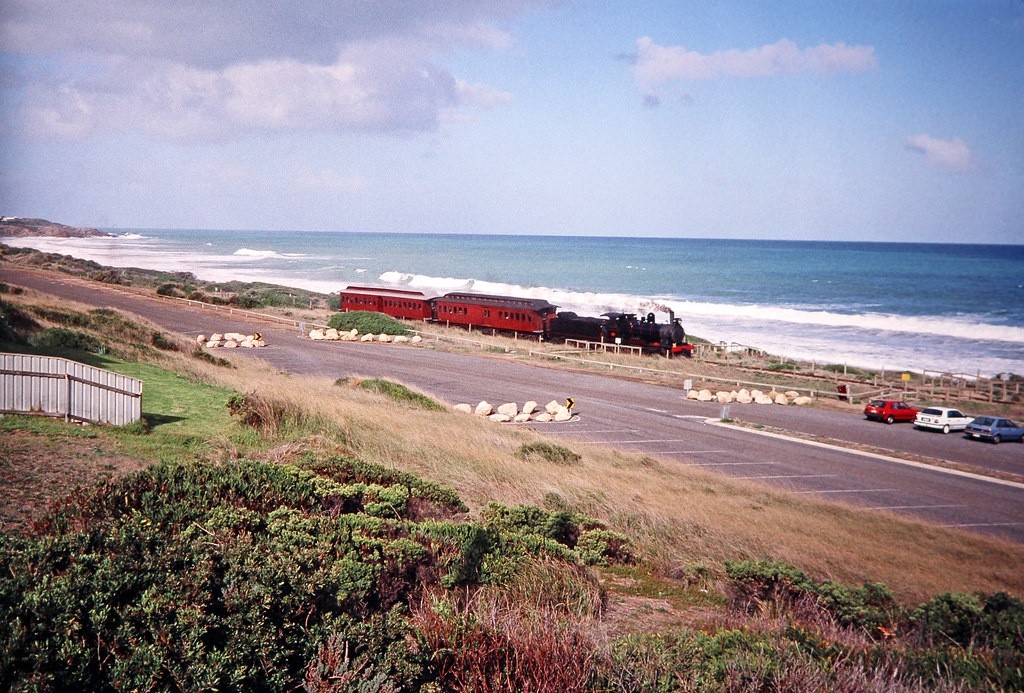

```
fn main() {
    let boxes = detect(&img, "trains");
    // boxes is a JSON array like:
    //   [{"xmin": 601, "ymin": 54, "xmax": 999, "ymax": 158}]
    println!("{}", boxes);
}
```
[{"xmin": 339, "ymin": 285, "xmax": 696, "ymax": 360}]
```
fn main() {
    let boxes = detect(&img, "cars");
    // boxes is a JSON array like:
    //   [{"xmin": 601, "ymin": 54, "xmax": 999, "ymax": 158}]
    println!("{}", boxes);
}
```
[
  {"xmin": 864, "ymin": 398, "xmax": 923, "ymax": 425},
  {"xmin": 964, "ymin": 415, "xmax": 1024, "ymax": 445},
  {"xmin": 914, "ymin": 406, "xmax": 975, "ymax": 435}
]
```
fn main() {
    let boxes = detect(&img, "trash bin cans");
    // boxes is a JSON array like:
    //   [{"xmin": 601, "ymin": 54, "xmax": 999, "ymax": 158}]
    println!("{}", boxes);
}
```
[{"xmin": 837, "ymin": 385, "xmax": 847, "ymax": 400}]
